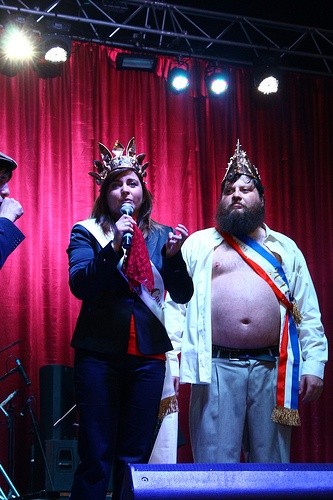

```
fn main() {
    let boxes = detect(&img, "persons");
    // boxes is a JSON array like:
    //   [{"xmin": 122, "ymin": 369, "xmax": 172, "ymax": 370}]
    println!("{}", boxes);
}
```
[
  {"xmin": 66, "ymin": 138, "xmax": 194, "ymax": 500},
  {"xmin": 161, "ymin": 139, "xmax": 328, "ymax": 462},
  {"xmin": 0, "ymin": 152, "xmax": 25, "ymax": 270}
]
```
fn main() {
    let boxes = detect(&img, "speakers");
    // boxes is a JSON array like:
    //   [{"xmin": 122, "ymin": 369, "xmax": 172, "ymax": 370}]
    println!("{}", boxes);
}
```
[
  {"xmin": 38, "ymin": 363, "xmax": 78, "ymax": 491},
  {"xmin": 118, "ymin": 462, "xmax": 333, "ymax": 500}
]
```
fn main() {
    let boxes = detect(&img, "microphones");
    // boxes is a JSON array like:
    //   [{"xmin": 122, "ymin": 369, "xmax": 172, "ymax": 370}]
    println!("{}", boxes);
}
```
[
  {"xmin": 120, "ymin": 202, "xmax": 136, "ymax": 248},
  {"xmin": 20, "ymin": 395, "xmax": 34, "ymax": 416},
  {"xmin": 15, "ymin": 357, "xmax": 31, "ymax": 384}
]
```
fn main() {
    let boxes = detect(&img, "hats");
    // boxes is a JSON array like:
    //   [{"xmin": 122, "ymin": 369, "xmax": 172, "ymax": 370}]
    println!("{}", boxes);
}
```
[
  {"xmin": 88, "ymin": 137, "xmax": 150, "ymax": 182},
  {"xmin": 0, "ymin": 153, "xmax": 18, "ymax": 170},
  {"xmin": 221, "ymin": 139, "xmax": 260, "ymax": 184}
]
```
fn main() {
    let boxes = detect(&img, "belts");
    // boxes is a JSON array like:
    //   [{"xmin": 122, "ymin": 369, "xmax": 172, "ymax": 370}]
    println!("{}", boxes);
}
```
[{"xmin": 209, "ymin": 348, "xmax": 281, "ymax": 363}]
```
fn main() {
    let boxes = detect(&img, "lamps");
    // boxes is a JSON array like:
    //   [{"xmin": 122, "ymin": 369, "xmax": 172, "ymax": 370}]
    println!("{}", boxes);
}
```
[
  {"xmin": 204, "ymin": 64, "xmax": 230, "ymax": 96},
  {"xmin": 40, "ymin": 34, "xmax": 71, "ymax": 63},
  {"xmin": 253, "ymin": 68, "xmax": 279, "ymax": 96},
  {"xmin": 166, "ymin": 59, "xmax": 191, "ymax": 94}
]
